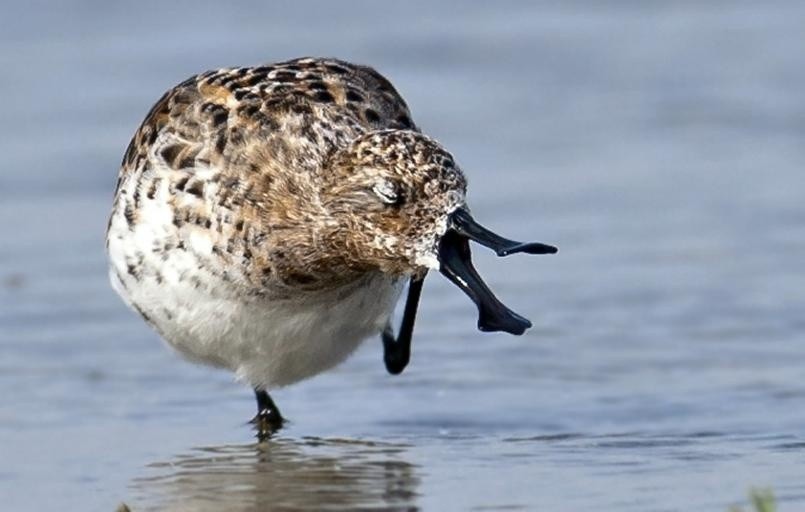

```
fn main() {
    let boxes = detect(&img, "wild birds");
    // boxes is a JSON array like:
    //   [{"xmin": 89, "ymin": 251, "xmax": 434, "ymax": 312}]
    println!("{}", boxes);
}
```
[{"xmin": 108, "ymin": 57, "xmax": 557, "ymax": 422}]
